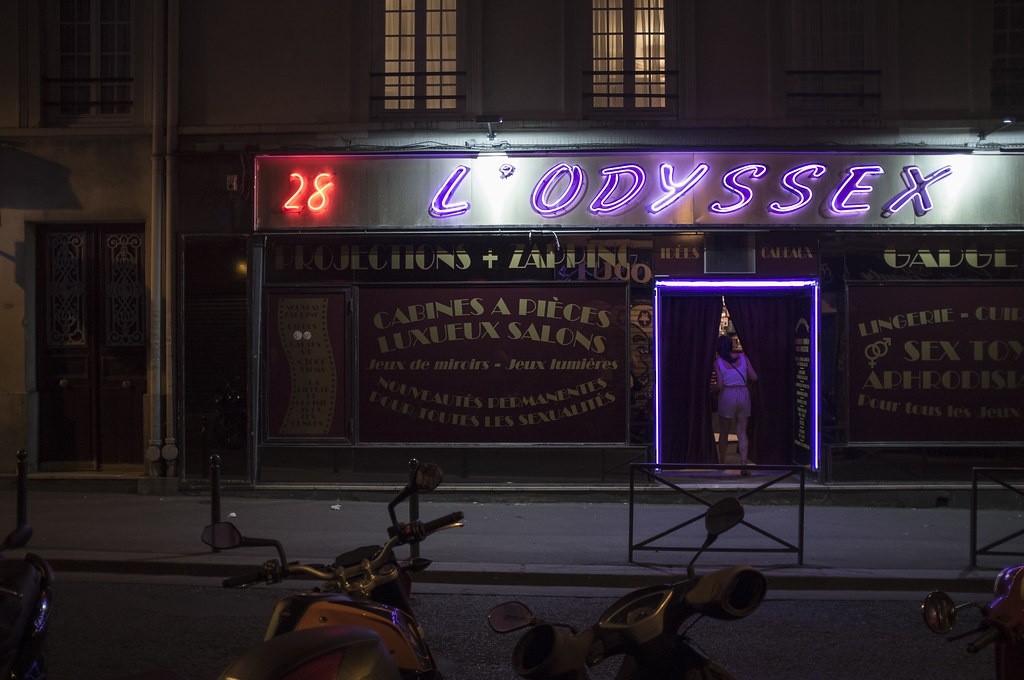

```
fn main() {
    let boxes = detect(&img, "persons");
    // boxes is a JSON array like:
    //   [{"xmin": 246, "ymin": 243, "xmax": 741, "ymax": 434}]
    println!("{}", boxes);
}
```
[{"xmin": 711, "ymin": 335, "xmax": 758, "ymax": 476}]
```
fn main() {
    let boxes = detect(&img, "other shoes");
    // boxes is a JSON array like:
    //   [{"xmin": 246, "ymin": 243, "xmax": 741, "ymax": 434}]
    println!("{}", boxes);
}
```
[{"xmin": 741, "ymin": 470, "xmax": 751, "ymax": 475}]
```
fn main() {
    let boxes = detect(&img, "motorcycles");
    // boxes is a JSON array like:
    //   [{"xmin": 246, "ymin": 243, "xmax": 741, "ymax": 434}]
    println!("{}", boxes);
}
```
[
  {"xmin": 488, "ymin": 497, "xmax": 767, "ymax": 680},
  {"xmin": 201, "ymin": 462, "xmax": 465, "ymax": 680},
  {"xmin": 0, "ymin": 525, "xmax": 56, "ymax": 680}
]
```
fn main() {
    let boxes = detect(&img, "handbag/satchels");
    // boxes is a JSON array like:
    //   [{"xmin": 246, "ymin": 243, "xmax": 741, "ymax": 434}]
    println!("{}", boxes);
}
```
[{"xmin": 746, "ymin": 382, "xmax": 753, "ymax": 392}]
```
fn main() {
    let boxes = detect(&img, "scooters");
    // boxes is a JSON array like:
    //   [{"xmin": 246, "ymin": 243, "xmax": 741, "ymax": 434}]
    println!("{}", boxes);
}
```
[{"xmin": 920, "ymin": 565, "xmax": 1024, "ymax": 680}]
